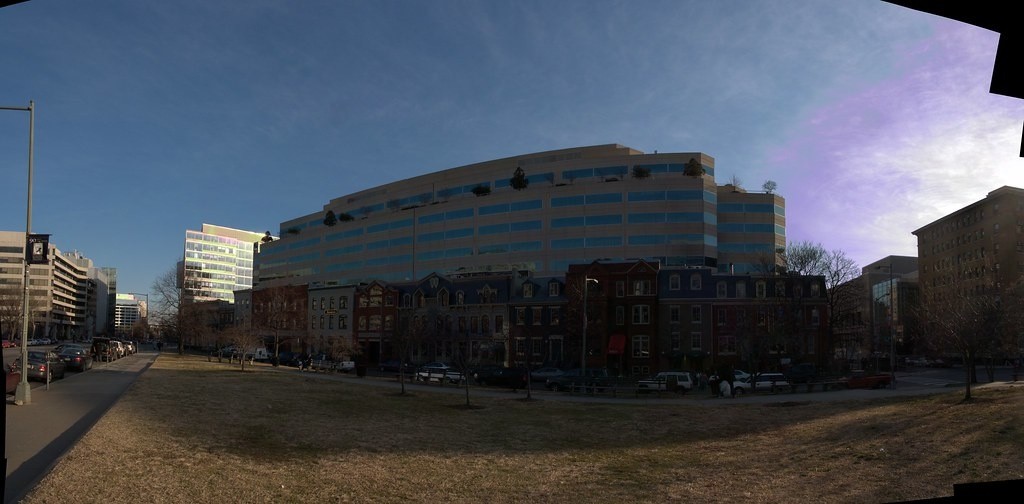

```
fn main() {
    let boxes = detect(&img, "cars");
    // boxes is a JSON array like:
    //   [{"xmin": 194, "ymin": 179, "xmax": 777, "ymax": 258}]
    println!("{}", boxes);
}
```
[
  {"xmin": 58, "ymin": 348, "xmax": 94, "ymax": 372},
  {"xmin": 10, "ymin": 349, "xmax": 67, "ymax": 384},
  {"xmin": 3, "ymin": 362, "xmax": 21, "ymax": 394},
  {"xmin": 470, "ymin": 361, "xmax": 609, "ymax": 394},
  {"xmin": 220, "ymin": 345, "xmax": 272, "ymax": 362},
  {"xmin": 51, "ymin": 343, "xmax": 86, "ymax": 355},
  {"xmin": 1, "ymin": 337, "xmax": 58, "ymax": 349}
]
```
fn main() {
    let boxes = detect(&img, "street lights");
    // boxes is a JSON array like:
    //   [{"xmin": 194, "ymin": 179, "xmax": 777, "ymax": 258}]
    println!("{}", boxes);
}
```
[
  {"xmin": 873, "ymin": 292, "xmax": 890, "ymax": 329},
  {"xmin": 580, "ymin": 274, "xmax": 600, "ymax": 394},
  {"xmin": 874, "ymin": 263, "xmax": 893, "ymax": 375}
]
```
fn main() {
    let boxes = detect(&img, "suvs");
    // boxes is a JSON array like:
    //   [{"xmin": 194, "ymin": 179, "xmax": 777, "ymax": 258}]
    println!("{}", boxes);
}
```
[
  {"xmin": 122, "ymin": 341, "xmax": 136, "ymax": 355},
  {"xmin": 414, "ymin": 362, "xmax": 466, "ymax": 384},
  {"xmin": 90, "ymin": 336, "xmax": 114, "ymax": 363},
  {"xmin": 708, "ymin": 370, "xmax": 789, "ymax": 396},
  {"xmin": 109, "ymin": 340, "xmax": 126, "ymax": 359},
  {"xmin": 635, "ymin": 371, "xmax": 694, "ymax": 396}
]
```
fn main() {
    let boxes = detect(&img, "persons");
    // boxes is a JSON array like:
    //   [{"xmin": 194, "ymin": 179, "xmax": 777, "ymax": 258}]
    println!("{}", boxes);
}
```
[
  {"xmin": 696, "ymin": 367, "xmax": 707, "ymax": 392},
  {"xmin": 718, "ymin": 376, "xmax": 731, "ymax": 399},
  {"xmin": 305, "ymin": 353, "xmax": 313, "ymax": 371}
]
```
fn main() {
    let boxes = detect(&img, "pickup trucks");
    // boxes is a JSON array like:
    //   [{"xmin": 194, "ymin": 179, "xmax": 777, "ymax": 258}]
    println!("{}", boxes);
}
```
[{"xmin": 310, "ymin": 353, "xmax": 355, "ymax": 373}]
